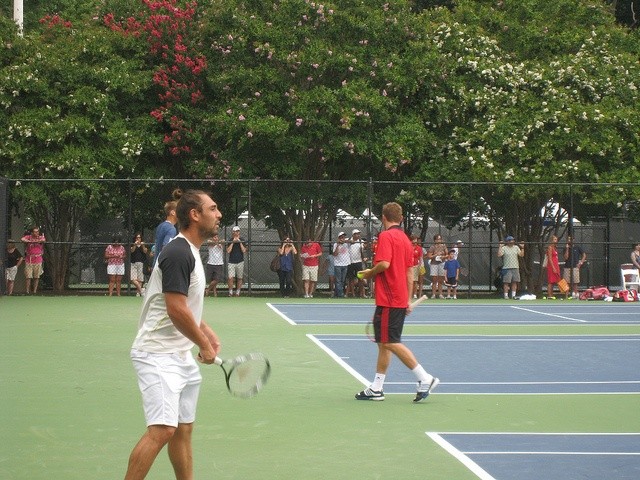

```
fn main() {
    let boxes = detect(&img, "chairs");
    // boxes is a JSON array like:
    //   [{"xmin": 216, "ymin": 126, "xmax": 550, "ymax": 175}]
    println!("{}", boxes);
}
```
[{"xmin": 621, "ymin": 269, "xmax": 640, "ymax": 290}]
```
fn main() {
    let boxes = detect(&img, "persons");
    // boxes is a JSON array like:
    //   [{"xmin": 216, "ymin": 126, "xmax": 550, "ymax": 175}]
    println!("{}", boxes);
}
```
[
  {"xmin": 21, "ymin": 226, "xmax": 46, "ymax": 296},
  {"xmin": 298, "ymin": 238, "xmax": 323, "ymax": 299},
  {"xmin": 226, "ymin": 225, "xmax": 248, "ymax": 297},
  {"xmin": 346, "ymin": 228, "xmax": 369, "ymax": 299},
  {"xmin": 276, "ymin": 235, "xmax": 298, "ymax": 298},
  {"xmin": 360, "ymin": 238, "xmax": 368, "ymax": 297},
  {"xmin": 355, "ymin": 201, "xmax": 442, "ymax": 402},
  {"xmin": 546, "ymin": 235, "xmax": 561, "ymax": 299},
  {"xmin": 332, "ymin": 232, "xmax": 351, "ymax": 299},
  {"xmin": 129, "ymin": 232, "xmax": 148, "ymax": 297},
  {"xmin": 562, "ymin": 234, "xmax": 586, "ymax": 301},
  {"xmin": 408, "ymin": 235, "xmax": 423, "ymax": 299},
  {"xmin": 151, "ymin": 201, "xmax": 181, "ymax": 268},
  {"xmin": 204, "ymin": 232, "xmax": 225, "ymax": 298},
  {"xmin": 417, "ymin": 238, "xmax": 428, "ymax": 299},
  {"xmin": 0, "ymin": 238, "xmax": 23, "ymax": 296},
  {"xmin": 443, "ymin": 250, "xmax": 461, "ymax": 300},
  {"xmin": 343, "ymin": 237, "xmax": 351, "ymax": 297},
  {"xmin": 124, "ymin": 188, "xmax": 223, "ymax": 480},
  {"xmin": 321, "ymin": 247, "xmax": 337, "ymax": 292},
  {"xmin": 428, "ymin": 234, "xmax": 449, "ymax": 300},
  {"xmin": 371, "ymin": 233, "xmax": 379, "ymax": 299},
  {"xmin": 451, "ymin": 240, "xmax": 464, "ymax": 260},
  {"xmin": 630, "ymin": 241, "xmax": 640, "ymax": 293},
  {"xmin": 149, "ymin": 245, "xmax": 156, "ymax": 259},
  {"xmin": 498, "ymin": 236, "xmax": 524, "ymax": 300},
  {"xmin": 103, "ymin": 237, "xmax": 127, "ymax": 297}
]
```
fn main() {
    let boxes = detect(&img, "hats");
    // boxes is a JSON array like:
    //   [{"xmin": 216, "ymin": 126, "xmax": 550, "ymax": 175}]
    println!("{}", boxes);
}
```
[
  {"xmin": 505, "ymin": 236, "xmax": 515, "ymax": 241},
  {"xmin": 434, "ymin": 234, "xmax": 443, "ymax": 241},
  {"xmin": 233, "ymin": 226, "xmax": 241, "ymax": 231},
  {"xmin": 352, "ymin": 229, "xmax": 361, "ymax": 235},
  {"xmin": 338, "ymin": 232, "xmax": 347, "ymax": 238}
]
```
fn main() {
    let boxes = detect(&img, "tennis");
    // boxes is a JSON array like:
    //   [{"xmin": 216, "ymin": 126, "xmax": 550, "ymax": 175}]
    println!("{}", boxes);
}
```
[{"xmin": 356, "ymin": 271, "xmax": 365, "ymax": 279}]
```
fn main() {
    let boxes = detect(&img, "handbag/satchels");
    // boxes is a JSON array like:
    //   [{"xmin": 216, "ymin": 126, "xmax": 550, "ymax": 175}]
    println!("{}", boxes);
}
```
[
  {"xmin": 558, "ymin": 279, "xmax": 570, "ymax": 293},
  {"xmin": 543, "ymin": 254, "xmax": 549, "ymax": 268},
  {"xmin": 613, "ymin": 289, "xmax": 637, "ymax": 302}
]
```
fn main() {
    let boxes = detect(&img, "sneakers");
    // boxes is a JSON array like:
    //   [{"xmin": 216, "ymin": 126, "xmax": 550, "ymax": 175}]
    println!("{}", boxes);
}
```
[
  {"xmin": 439, "ymin": 294, "xmax": 445, "ymax": 299},
  {"xmin": 431, "ymin": 295, "xmax": 436, "ymax": 299},
  {"xmin": 412, "ymin": 375, "xmax": 439, "ymax": 402},
  {"xmin": 414, "ymin": 294, "xmax": 418, "ymax": 299},
  {"xmin": 354, "ymin": 387, "xmax": 386, "ymax": 401}
]
